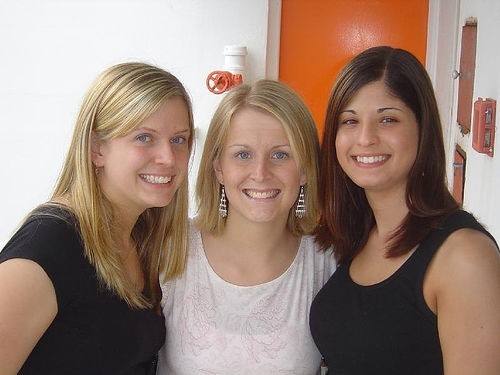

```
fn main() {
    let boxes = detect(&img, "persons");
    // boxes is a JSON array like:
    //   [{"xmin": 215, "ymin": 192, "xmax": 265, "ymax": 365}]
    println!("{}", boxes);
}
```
[
  {"xmin": 0, "ymin": 63, "xmax": 193, "ymax": 375},
  {"xmin": 310, "ymin": 46, "xmax": 500, "ymax": 375},
  {"xmin": 156, "ymin": 80, "xmax": 338, "ymax": 375}
]
